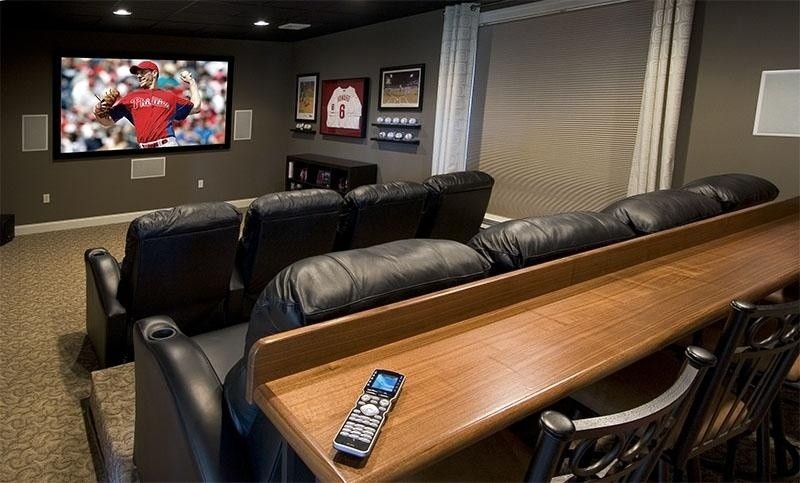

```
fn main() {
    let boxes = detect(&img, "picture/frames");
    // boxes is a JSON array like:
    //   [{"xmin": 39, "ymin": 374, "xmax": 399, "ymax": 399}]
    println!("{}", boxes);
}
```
[
  {"xmin": 295, "ymin": 71, "xmax": 319, "ymax": 124},
  {"xmin": 377, "ymin": 62, "xmax": 426, "ymax": 113},
  {"xmin": 319, "ymin": 77, "xmax": 370, "ymax": 139}
]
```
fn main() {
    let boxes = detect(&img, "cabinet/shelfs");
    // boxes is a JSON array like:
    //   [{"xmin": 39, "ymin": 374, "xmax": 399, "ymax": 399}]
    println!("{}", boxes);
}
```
[
  {"xmin": 371, "ymin": 121, "xmax": 422, "ymax": 146},
  {"xmin": 284, "ymin": 152, "xmax": 379, "ymax": 197}
]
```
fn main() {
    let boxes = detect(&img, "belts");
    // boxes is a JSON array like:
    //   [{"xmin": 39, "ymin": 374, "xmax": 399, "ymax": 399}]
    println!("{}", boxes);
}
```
[{"xmin": 141, "ymin": 138, "xmax": 168, "ymax": 147}]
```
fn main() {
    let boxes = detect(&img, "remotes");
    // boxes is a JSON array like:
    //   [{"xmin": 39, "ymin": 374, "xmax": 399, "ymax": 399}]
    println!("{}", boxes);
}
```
[{"xmin": 332, "ymin": 368, "xmax": 406, "ymax": 458}]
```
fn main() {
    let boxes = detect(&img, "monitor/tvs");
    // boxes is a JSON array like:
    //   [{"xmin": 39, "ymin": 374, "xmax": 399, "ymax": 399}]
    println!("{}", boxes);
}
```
[{"xmin": 52, "ymin": 43, "xmax": 234, "ymax": 161}]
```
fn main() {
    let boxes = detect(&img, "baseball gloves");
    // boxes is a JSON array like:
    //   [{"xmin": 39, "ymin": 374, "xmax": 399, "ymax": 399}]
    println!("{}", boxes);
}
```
[{"xmin": 95, "ymin": 88, "xmax": 120, "ymax": 118}]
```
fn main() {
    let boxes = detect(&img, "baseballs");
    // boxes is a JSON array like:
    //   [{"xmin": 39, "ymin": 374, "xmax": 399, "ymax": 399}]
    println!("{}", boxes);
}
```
[
  {"xmin": 182, "ymin": 71, "xmax": 189, "ymax": 77},
  {"xmin": 376, "ymin": 117, "xmax": 416, "ymax": 124},
  {"xmin": 378, "ymin": 132, "xmax": 412, "ymax": 139}
]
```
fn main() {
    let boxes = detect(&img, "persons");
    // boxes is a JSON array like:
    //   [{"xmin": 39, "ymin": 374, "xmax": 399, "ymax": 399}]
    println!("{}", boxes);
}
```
[{"xmin": 61, "ymin": 58, "xmax": 228, "ymax": 152}]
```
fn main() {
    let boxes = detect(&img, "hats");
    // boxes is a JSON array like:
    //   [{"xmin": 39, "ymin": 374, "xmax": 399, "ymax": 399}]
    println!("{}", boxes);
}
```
[{"xmin": 129, "ymin": 61, "xmax": 160, "ymax": 79}]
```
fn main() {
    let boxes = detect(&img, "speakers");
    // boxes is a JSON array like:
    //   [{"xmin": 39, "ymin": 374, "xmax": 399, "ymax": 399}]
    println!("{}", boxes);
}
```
[
  {"xmin": 22, "ymin": 114, "xmax": 49, "ymax": 152},
  {"xmin": 234, "ymin": 110, "xmax": 252, "ymax": 141},
  {"xmin": 131, "ymin": 156, "xmax": 166, "ymax": 180}
]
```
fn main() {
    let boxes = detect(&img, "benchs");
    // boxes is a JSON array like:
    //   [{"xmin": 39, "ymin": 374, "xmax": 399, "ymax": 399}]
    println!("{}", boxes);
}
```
[
  {"xmin": 127, "ymin": 170, "xmax": 798, "ymax": 482},
  {"xmin": 82, "ymin": 170, "xmax": 497, "ymax": 366}
]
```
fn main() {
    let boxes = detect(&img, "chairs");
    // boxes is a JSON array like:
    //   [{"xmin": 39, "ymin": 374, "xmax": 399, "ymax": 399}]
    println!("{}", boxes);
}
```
[
  {"xmin": 388, "ymin": 344, "xmax": 720, "ymax": 482},
  {"xmin": 702, "ymin": 314, "xmax": 798, "ymax": 482},
  {"xmin": 571, "ymin": 299, "xmax": 800, "ymax": 483}
]
